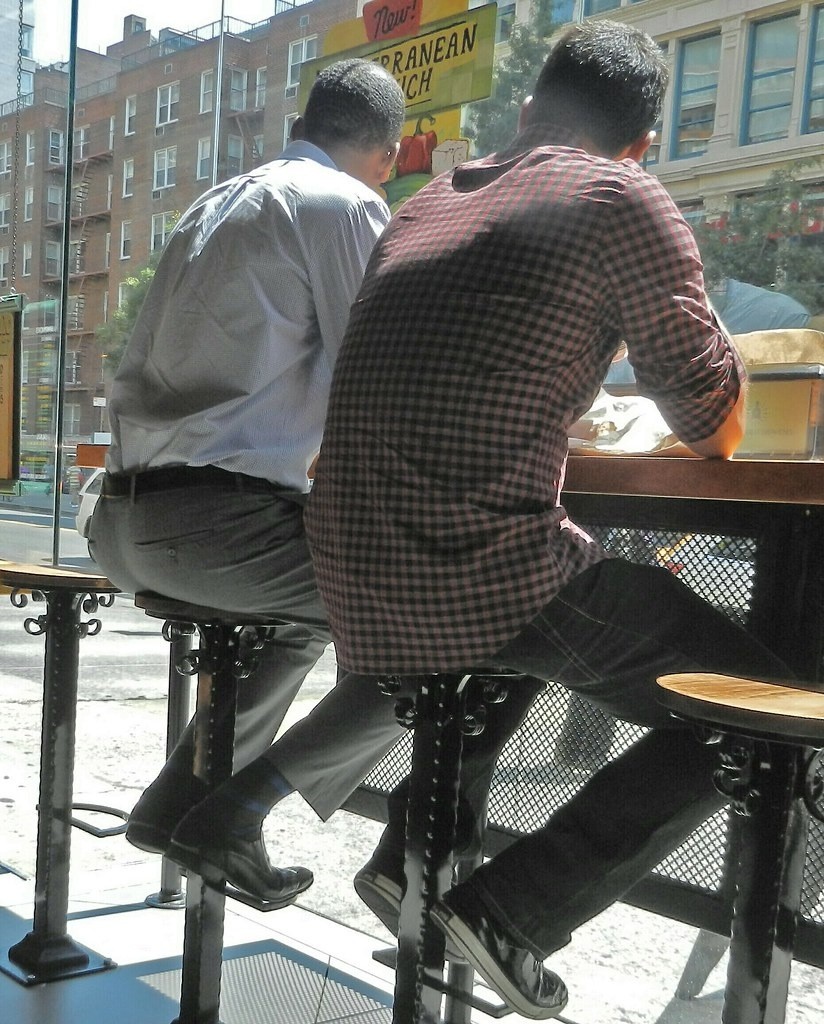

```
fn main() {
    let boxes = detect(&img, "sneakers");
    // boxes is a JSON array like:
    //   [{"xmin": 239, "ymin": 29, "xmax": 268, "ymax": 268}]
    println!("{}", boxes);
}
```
[
  {"xmin": 354, "ymin": 828, "xmax": 408, "ymax": 937},
  {"xmin": 429, "ymin": 883, "xmax": 569, "ymax": 1020}
]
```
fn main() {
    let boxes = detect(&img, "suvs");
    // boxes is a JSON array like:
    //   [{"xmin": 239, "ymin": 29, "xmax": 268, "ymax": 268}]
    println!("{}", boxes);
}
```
[{"xmin": 75, "ymin": 469, "xmax": 106, "ymax": 563}]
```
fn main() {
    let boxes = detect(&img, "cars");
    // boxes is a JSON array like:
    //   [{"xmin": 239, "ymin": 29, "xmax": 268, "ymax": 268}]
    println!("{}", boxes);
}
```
[{"xmin": 657, "ymin": 531, "xmax": 753, "ymax": 609}]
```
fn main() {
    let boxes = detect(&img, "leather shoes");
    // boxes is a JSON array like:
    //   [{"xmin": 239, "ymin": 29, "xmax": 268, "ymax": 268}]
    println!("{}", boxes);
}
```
[
  {"xmin": 166, "ymin": 805, "xmax": 314, "ymax": 902},
  {"xmin": 125, "ymin": 757, "xmax": 205, "ymax": 857}
]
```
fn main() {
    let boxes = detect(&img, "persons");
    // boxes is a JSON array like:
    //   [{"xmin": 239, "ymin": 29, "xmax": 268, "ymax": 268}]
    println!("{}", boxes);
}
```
[
  {"xmin": 603, "ymin": 254, "xmax": 810, "ymax": 385},
  {"xmin": 304, "ymin": 18, "xmax": 802, "ymax": 1020},
  {"xmin": 86, "ymin": 60, "xmax": 426, "ymax": 909}
]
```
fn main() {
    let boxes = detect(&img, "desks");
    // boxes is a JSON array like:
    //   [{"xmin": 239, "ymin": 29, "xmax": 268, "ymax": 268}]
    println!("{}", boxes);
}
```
[{"xmin": 559, "ymin": 455, "xmax": 824, "ymax": 647}]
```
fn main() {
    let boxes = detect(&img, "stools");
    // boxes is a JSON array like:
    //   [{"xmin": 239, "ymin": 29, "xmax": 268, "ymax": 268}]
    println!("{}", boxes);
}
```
[
  {"xmin": 0, "ymin": 565, "xmax": 131, "ymax": 988},
  {"xmin": 378, "ymin": 668, "xmax": 549, "ymax": 1024},
  {"xmin": 650, "ymin": 668, "xmax": 824, "ymax": 1024},
  {"xmin": 133, "ymin": 590, "xmax": 298, "ymax": 1024}
]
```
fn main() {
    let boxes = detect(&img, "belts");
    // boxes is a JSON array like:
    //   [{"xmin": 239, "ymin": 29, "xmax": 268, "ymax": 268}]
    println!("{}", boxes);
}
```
[{"xmin": 100, "ymin": 463, "xmax": 277, "ymax": 497}]
